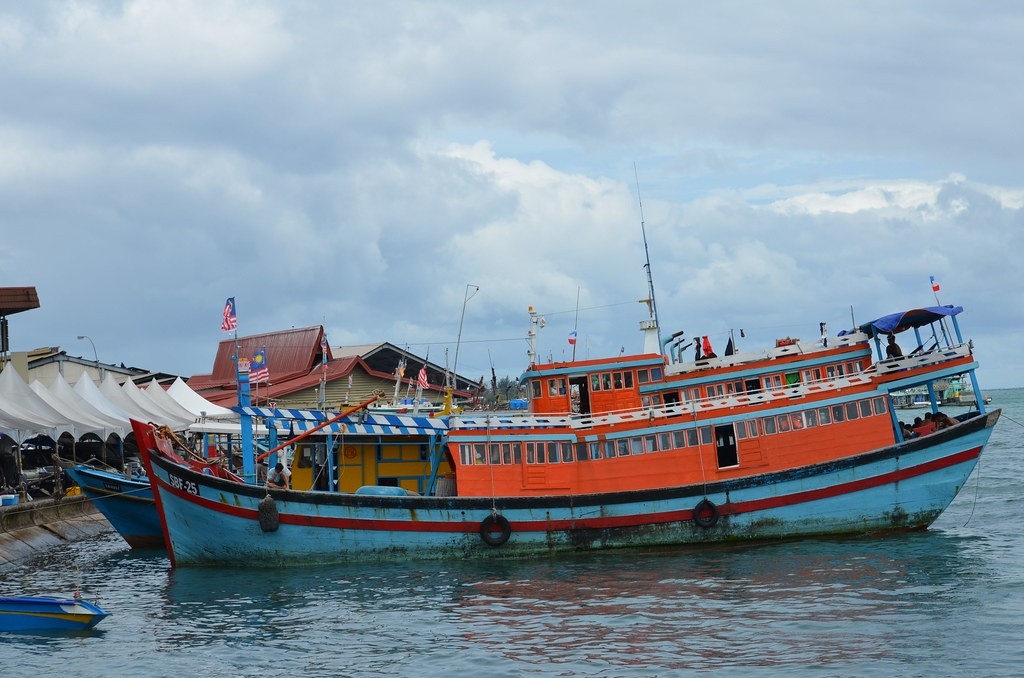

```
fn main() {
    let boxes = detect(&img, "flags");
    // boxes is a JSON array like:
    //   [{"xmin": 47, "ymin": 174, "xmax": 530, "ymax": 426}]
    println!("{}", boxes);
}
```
[
  {"xmin": 221, "ymin": 298, "xmax": 237, "ymax": 334},
  {"xmin": 249, "ymin": 347, "xmax": 269, "ymax": 383},
  {"xmin": 321, "ymin": 331, "xmax": 328, "ymax": 371},
  {"xmin": 930, "ymin": 276, "xmax": 940, "ymax": 293},
  {"xmin": 418, "ymin": 367, "xmax": 430, "ymax": 390},
  {"xmin": 568, "ymin": 331, "xmax": 577, "ymax": 344}
]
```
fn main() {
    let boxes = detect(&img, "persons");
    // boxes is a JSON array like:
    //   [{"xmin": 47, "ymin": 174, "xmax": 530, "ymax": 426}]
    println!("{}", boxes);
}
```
[
  {"xmin": 899, "ymin": 412, "xmax": 960, "ymax": 441},
  {"xmin": 256, "ymin": 459, "xmax": 267, "ymax": 486},
  {"xmin": 886, "ymin": 335, "xmax": 905, "ymax": 362},
  {"xmin": 266, "ymin": 462, "xmax": 291, "ymax": 490}
]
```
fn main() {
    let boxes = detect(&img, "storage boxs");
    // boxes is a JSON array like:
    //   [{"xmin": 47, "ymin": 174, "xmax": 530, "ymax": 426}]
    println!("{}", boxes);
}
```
[
  {"xmin": 66, "ymin": 486, "xmax": 81, "ymax": 496},
  {"xmin": 1, "ymin": 494, "xmax": 20, "ymax": 507}
]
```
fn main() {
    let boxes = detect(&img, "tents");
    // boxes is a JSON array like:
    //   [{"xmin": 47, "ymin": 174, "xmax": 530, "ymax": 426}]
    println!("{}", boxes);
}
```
[{"xmin": 0, "ymin": 360, "xmax": 239, "ymax": 489}]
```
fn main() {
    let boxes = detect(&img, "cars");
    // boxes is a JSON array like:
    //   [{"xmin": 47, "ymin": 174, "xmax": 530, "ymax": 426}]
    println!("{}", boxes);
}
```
[{"xmin": 123, "ymin": 456, "xmax": 144, "ymax": 475}]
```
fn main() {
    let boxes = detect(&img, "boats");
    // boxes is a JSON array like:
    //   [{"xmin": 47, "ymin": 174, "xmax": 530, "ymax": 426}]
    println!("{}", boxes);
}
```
[
  {"xmin": 0, "ymin": 592, "xmax": 112, "ymax": 635},
  {"xmin": 54, "ymin": 164, "xmax": 1003, "ymax": 552}
]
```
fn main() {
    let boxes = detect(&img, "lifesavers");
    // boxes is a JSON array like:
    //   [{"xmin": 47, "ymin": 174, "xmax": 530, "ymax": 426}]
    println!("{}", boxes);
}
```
[
  {"xmin": 478, "ymin": 513, "xmax": 511, "ymax": 546},
  {"xmin": 693, "ymin": 499, "xmax": 720, "ymax": 528}
]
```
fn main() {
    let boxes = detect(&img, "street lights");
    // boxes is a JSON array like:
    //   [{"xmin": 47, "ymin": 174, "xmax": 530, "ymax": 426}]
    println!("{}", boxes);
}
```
[{"xmin": 75, "ymin": 334, "xmax": 103, "ymax": 384}]
